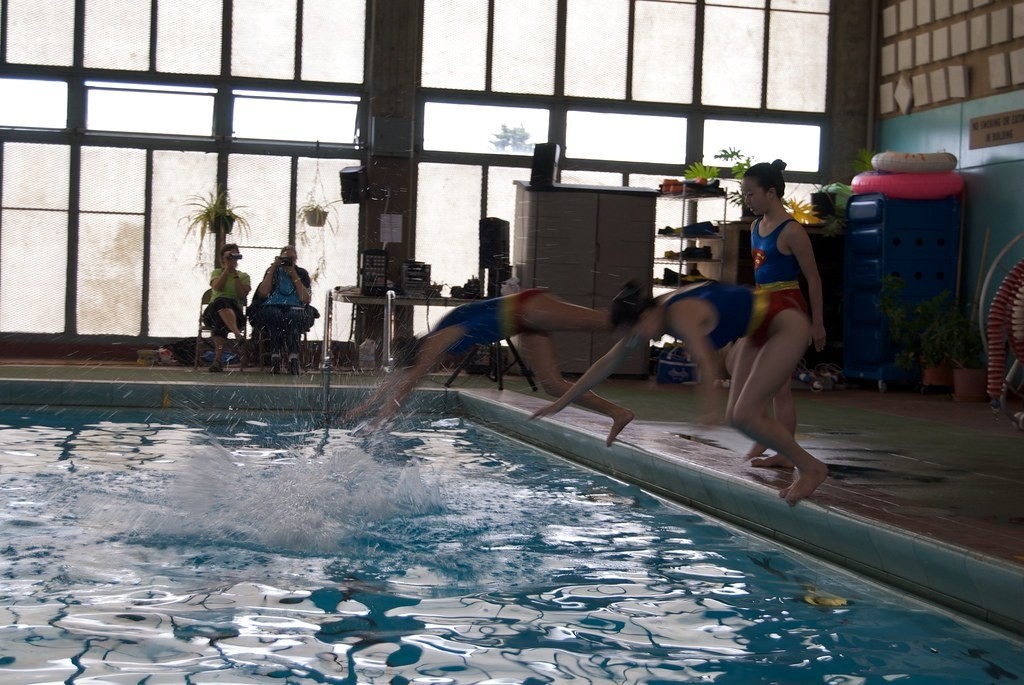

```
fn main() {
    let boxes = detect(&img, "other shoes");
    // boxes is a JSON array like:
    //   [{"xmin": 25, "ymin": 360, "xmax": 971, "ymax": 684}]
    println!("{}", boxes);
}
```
[
  {"xmin": 658, "ymin": 173, "xmax": 725, "ymax": 287},
  {"xmin": 269, "ymin": 354, "xmax": 300, "ymax": 375},
  {"xmin": 207, "ymin": 360, "xmax": 222, "ymax": 372},
  {"xmin": 236, "ymin": 337, "xmax": 251, "ymax": 354}
]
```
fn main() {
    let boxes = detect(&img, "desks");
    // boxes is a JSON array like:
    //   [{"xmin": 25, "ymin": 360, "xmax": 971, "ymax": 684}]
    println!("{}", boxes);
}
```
[{"xmin": 318, "ymin": 286, "xmax": 485, "ymax": 375}]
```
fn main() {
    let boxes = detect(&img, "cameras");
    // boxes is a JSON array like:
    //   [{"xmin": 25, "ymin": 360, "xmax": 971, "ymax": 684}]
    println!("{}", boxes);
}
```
[
  {"xmin": 278, "ymin": 256, "xmax": 293, "ymax": 266},
  {"xmin": 227, "ymin": 253, "xmax": 242, "ymax": 260}
]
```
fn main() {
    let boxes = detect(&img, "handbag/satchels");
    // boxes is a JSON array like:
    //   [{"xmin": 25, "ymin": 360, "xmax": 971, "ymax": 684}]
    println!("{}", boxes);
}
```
[{"xmin": 655, "ymin": 343, "xmax": 698, "ymax": 385}]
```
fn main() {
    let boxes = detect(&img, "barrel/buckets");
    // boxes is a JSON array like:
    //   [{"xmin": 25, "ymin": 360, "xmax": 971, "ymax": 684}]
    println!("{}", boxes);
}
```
[{"xmin": 358, "ymin": 338, "xmax": 376, "ymax": 371}]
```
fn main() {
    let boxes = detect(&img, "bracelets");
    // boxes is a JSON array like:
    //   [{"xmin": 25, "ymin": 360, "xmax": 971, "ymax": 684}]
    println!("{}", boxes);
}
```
[
  {"xmin": 233, "ymin": 276, "xmax": 239, "ymax": 279},
  {"xmin": 292, "ymin": 277, "xmax": 301, "ymax": 283}
]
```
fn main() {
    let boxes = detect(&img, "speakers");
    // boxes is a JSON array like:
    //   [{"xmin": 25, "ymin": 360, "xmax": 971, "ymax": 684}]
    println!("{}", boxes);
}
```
[
  {"xmin": 479, "ymin": 218, "xmax": 510, "ymax": 270},
  {"xmin": 339, "ymin": 165, "xmax": 368, "ymax": 204},
  {"xmin": 530, "ymin": 143, "xmax": 561, "ymax": 187}
]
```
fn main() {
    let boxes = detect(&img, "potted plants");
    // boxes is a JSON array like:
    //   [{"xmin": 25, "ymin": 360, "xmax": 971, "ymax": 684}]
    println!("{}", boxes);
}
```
[
  {"xmin": 180, "ymin": 183, "xmax": 249, "ymax": 271},
  {"xmin": 292, "ymin": 193, "xmax": 348, "ymax": 248},
  {"xmin": 684, "ymin": 162, "xmax": 719, "ymax": 183},
  {"xmin": 879, "ymin": 272, "xmax": 991, "ymax": 402}
]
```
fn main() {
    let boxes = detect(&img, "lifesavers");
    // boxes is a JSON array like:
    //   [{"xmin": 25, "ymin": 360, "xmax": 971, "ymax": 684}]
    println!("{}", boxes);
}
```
[
  {"xmin": 850, "ymin": 172, "xmax": 964, "ymax": 199},
  {"xmin": 871, "ymin": 151, "xmax": 958, "ymax": 172}
]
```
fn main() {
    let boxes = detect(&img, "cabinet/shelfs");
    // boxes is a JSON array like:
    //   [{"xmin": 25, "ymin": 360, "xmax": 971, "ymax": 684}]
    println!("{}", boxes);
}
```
[{"xmin": 478, "ymin": 179, "xmax": 967, "ymax": 393}]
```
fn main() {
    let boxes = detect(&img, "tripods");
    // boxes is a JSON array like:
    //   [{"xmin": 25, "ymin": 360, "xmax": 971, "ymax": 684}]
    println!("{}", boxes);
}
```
[{"xmin": 446, "ymin": 269, "xmax": 537, "ymax": 393}]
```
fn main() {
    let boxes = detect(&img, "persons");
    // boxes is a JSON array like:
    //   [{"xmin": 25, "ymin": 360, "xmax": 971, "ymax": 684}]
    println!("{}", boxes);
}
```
[
  {"xmin": 253, "ymin": 244, "xmax": 311, "ymax": 377},
  {"xmin": 525, "ymin": 278, "xmax": 829, "ymax": 507},
  {"xmin": 724, "ymin": 159, "xmax": 826, "ymax": 471},
  {"xmin": 346, "ymin": 288, "xmax": 636, "ymax": 447},
  {"xmin": 202, "ymin": 243, "xmax": 251, "ymax": 372}
]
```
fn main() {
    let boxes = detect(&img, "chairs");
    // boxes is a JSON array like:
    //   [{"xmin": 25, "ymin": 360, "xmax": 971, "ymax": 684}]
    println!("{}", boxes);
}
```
[{"xmin": 194, "ymin": 289, "xmax": 307, "ymax": 374}]
